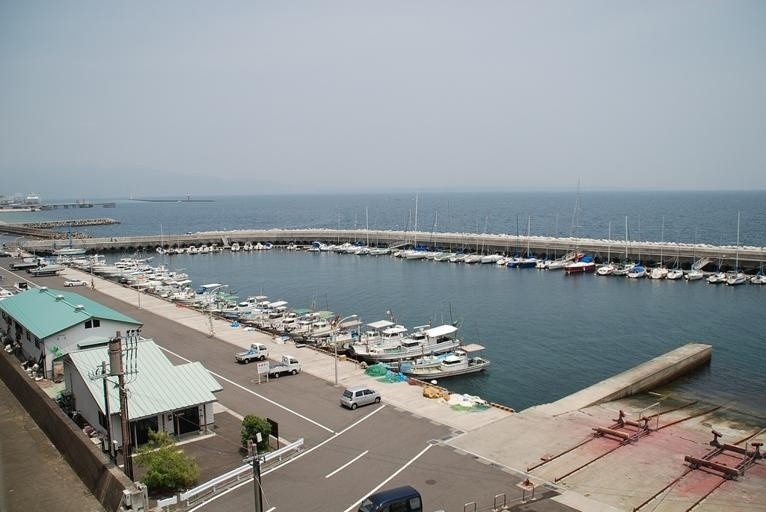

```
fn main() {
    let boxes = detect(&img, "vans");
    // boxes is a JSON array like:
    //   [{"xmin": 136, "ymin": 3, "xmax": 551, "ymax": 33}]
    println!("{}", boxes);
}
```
[
  {"xmin": 341, "ymin": 386, "xmax": 380, "ymax": 410},
  {"xmin": 358, "ymin": 485, "xmax": 423, "ymax": 511}
]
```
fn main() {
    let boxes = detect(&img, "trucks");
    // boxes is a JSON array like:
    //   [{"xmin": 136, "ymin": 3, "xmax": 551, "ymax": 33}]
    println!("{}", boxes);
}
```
[{"xmin": 9, "ymin": 257, "xmax": 47, "ymax": 269}]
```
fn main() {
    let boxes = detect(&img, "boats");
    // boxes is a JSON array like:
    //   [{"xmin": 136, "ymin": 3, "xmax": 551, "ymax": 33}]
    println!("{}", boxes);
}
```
[
  {"xmin": 53, "ymin": 255, "xmax": 190, "ymax": 297},
  {"xmin": 188, "ymin": 240, "xmax": 272, "ymax": 254},
  {"xmin": 51, "ymin": 247, "xmax": 85, "ymax": 254}
]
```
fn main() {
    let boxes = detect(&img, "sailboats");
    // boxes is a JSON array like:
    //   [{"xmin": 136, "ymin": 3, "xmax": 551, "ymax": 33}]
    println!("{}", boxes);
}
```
[
  {"xmin": 156, "ymin": 223, "xmax": 186, "ymax": 254},
  {"xmin": 598, "ymin": 211, "xmax": 766, "ymax": 285}
]
